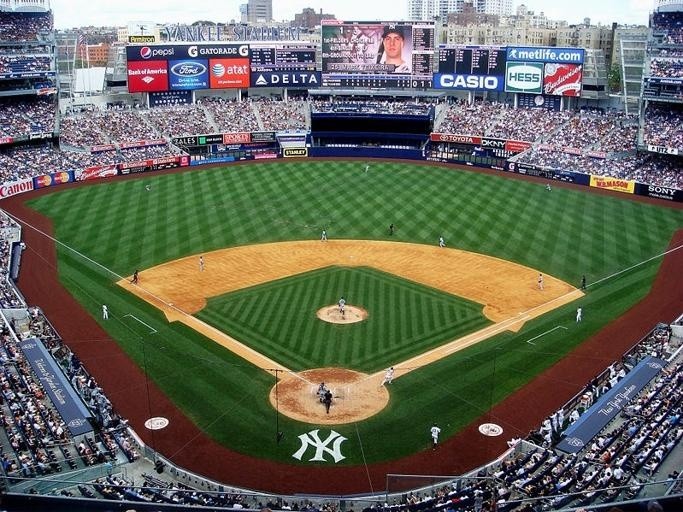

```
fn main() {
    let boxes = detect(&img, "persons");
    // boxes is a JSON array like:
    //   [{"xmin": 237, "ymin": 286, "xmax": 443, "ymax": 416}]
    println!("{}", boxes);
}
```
[
  {"xmin": 1, "ymin": 215, "xmax": 683, "ymax": 512},
  {"xmin": 0, "ymin": 14, "xmax": 682, "ymax": 191}
]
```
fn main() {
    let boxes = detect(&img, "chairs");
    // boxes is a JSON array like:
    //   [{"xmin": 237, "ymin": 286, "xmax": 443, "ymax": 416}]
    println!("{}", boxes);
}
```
[
  {"xmin": 55, "ymin": 97, "xmax": 638, "ymax": 187},
  {"xmin": 0, "ymin": 10, "xmax": 57, "ymax": 185},
  {"xmin": 537, "ymin": 323, "xmax": 682, "ymax": 511},
  {"xmin": 642, "ymin": 14, "xmax": 681, "ymax": 192},
  {"xmin": 0, "ymin": 206, "xmax": 137, "ymax": 511},
  {"xmin": 124, "ymin": 451, "xmax": 536, "ymax": 512}
]
